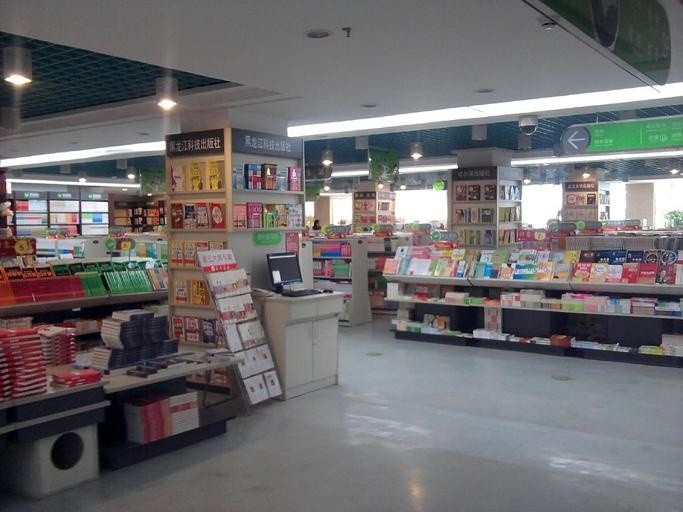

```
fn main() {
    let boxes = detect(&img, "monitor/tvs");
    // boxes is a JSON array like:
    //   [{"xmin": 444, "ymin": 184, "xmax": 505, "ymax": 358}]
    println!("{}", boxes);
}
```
[{"xmin": 266, "ymin": 252, "xmax": 303, "ymax": 293}]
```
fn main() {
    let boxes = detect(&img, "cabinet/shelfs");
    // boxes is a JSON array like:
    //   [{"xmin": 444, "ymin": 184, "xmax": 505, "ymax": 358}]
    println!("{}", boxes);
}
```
[
  {"xmin": 312, "ymin": 239, "xmax": 372, "ymax": 326},
  {"xmin": 447, "ymin": 179, "xmax": 524, "ymax": 246},
  {"xmin": 382, "ymin": 248, "xmax": 683, "ymax": 367},
  {"xmin": 165, "ymin": 155, "xmax": 226, "ymax": 395},
  {"xmin": 109, "ymin": 193, "xmax": 166, "ymax": 234},
  {"xmin": 351, "ymin": 198, "xmax": 395, "ymax": 227},
  {"xmin": 255, "ymin": 290, "xmax": 348, "ymax": 401},
  {"xmin": 14, "ymin": 190, "xmax": 109, "ymax": 236},
  {"xmin": 0, "ymin": 291, "xmax": 246, "ymax": 498},
  {"xmin": 358, "ymin": 232, "xmax": 415, "ymax": 315},
  {"xmin": 231, "ymin": 151, "xmax": 305, "ymax": 230}
]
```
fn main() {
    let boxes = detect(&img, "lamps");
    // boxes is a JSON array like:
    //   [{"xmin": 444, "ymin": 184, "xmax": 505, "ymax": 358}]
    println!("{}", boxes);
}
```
[
  {"xmin": 156, "ymin": 71, "xmax": 179, "ymax": 110},
  {"xmin": 410, "ymin": 131, "xmax": 423, "ymax": 162},
  {"xmin": 322, "ymin": 141, "xmax": 332, "ymax": 167},
  {"xmin": 2, "ymin": 46, "xmax": 32, "ymax": 86}
]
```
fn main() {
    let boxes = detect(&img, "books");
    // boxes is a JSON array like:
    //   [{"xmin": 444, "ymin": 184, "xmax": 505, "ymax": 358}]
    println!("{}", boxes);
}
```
[
  {"xmin": 184, "ymin": 203, "xmax": 196, "ymax": 229},
  {"xmin": 314, "ymin": 280, "xmax": 352, "ymax": 296},
  {"xmin": 186, "ymin": 316, "xmax": 200, "ymax": 344},
  {"xmin": 37, "ymin": 265, "xmax": 53, "ymax": 278},
  {"xmin": 571, "ymin": 262, "xmax": 591, "ymax": 282},
  {"xmin": 210, "ymin": 242, "xmax": 222, "ymax": 249},
  {"xmin": 366, "ymin": 234, "xmax": 400, "ymax": 252},
  {"xmin": 500, "ymin": 228, "xmax": 523, "ymax": 242},
  {"xmin": 204, "ymin": 319, "xmax": 217, "ymax": 343},
  {"xmin": 501, "ymin": 291, "xmax": 520, "ymax": 308},
  {"xmin": 566, "ymin": 193, "xmax": 576, "ymax": 205},
  {"xmin": 367, "ymin": 255, "xmax": 400, "ymax": 274},
  {"xmin": 265, "ymin": 164, "xmax": 277, "ymax": 190},
  {"xmin": 173, "ymin": 279, "xmax": 189, "ymax": 305},
  {"xmin": 564, "ymin": 250, "xmax": 582, "ymax": 266},
  {"xmin": 574, "ymin": 340, "xmax": 661, "ymax": 356},
  {"xmin": 550, "ymin": 250, "xmax": 562, "ymax": 263},
  {"xmin": 482, "ymin": 228, "xmax": 493, "ymax": 247},
  {"xmin": 459, "ymin": 332, "xmax": 473, "ymax": 339},
  {"xmin": 608, "ymin": 265, "xmax": 622, "ymax": 282},
  {"xmin": 311, "ymin": 241, "xmax": 351, "ymax": 256},
  {"xmin": 399, "ymin": 293, "xmax": 419, "ymax": 302},
  {"xmin": 172, "ymin": 315, "xmax": 184, "ymax": 340},
  {"xmin": 102, "ymin": 268, "xmax": 170, "ymax": 294},
  {"xmin": 453, "ymin": 230, "xmax": 465, "ymax": 245},
  {"xmin": 655, "ymin": 298, "xmax": 683, "ymax": 317},
  {"xmin": 422, "ymin": 313, "xmax": 460, "ymax": 335},
  {"xmin": 5, "ymin": 266, "xmax": 23, "ymax": 279},
  {"xmin": 454, "ymin": 209, "xmax": 466, "ymax": 226},
  {"xmin": 644, "ymin": 250, "xmax": 662, "ymax": 264},
  {"xmin": 500, "ymin": 185, "xmax": 522, "ymax": 199},
  {"xmin": 661, "ymin": 250, "xmax": 677, "ymax": 263},
  {"xmin": 562, "ymin": 293, "xmax": 609, "ymax": 313},
  {"xmin": 355, "ymin": 199, "xmax": 374, "ymax": 235},
  {"xmin": 369, "ymin": 277, "xmax": 388, "ymax": 312},
  {"xmin": 0, "ymin": 275, "xmax": 85, "ymax": 306},
  {"xmin": 75, "ymin": 271, "xmax": 107, "ymax": 298},
  {"xmin": 456, "ymin": 184, "xmax": 466, "ymax": 200},
  {"xmin": 196, "ymin": 241, "xmax": 208, "ymax": 266},
  {"xmin": 195, "ymin": 204, "xmax": 208, "ymax": 228},
  {"xmin": 612, "ymin": 251, "xmax": 626, "ymax": 265},
  {"xmin": 171, "ymin": 201, "xmax": 184, "ymax": 228},
  {"xmin": 249, "ymin": 163, "xmax": 262, "ymax": 189},
  {"xmin": 415, "ymin": 285, "xmax": 441, "ymax": 303},
  {"xmin": 341, "ymin": 300, "xmax": 350, "ymax": 322},
  {"xmin": 38, "ymin": 323, "xmax": 76, "ymax": 365},
  {"xmin": 0, "ymin": 318, "xmax": 48, "ymax": 401},
  {"xmin": 587, "ymin": 195, "xmax": 595, "ymax": 203},
  {"xmin": 232, "ymin": 164, "xmax": 245, "ymax": 191},
  {"xmin": 482, "ymin": 208, "xmax": 492, "ymax": 224},
  {"xmin": 552, "ymin": 264, "xmax": 572, "ymax": 281},
  {"xmin": 608, "ymin": 297, "xmax": 631, "ymax": 314},
  {"xmin": 232, "ymin": 204, "xmax": 247, "ymax": 229},
  {"xmin": 591, "ymin": 264, "xmax": 608, "ymax": 282},
  {"xmin": 581, "ymin": 249, "xmax": 595, "ymax": 263},
  {"xmin": 575, "ymin": 193, "xmax": 586, "ymax": 205},
  {"xmin": 623, "ymin": 263, "xmax": 639, "ymax": 284},
  {"xmin": 401, "ymin": 247, "xmax": 555, "ymax": 281},
  {"xmin": 468, "ymin": 185, "xmax": 480, "ymax": 200},
  {"xmin": 473, "ymin": 328, "xmax": 550, "ymax": 344},
  {"xmin": 541, "ymin": 298, "xmax": 561, "ymax": 310},
  {"xmin": 170, "ymin": 239, "xmax": 184, "ymax": 267},
  {"xmin": 91, "ymin": 339, "xmax": 180, "ymax": 369},
  {"xmin": 51, "ymin": 368, "xmax": 101, "ymax": 388},
  {"xmin": 565, "ymin": 236, "xmax": 683, "ymax": 250},
  {"xmin": 125, "ymin": 388, "xmax": 198, "ymax": 444},
  {"xmin": 212, "ymin": 271, "xmax": 257, "ymax": 323},
  {"xmin": 639, "ymin": 264, "xmax": 656, "ymax": 283},
  {"xmin": 184, "ymin": 241, "xmax": 195, "ymax": 265},
  {"xmin": 190, "ymin": 161, "xmax": 204, "ymax": 193},
  {"xmin": 209, "ymin": 204, "xmax": 226, "ymax": 229},
  {"xmin": 469, "ymin": 229, "xmax": 480, "ymax": 246},
  {"xmin": 386, "ymin": 282, "xmax": 398, "ymax": 301},
  {"xmin": 521, "ymin": 290, "xmax": 543, "ymax": 309},
  {"xmin": 222, "ymin": 320, "xmax": 283, "ymax": 406},
  {"xmin": 118, "ymin": 207, "xmax": 165, "ymax": 232},
  {"xmin": 73, "ymin": 319, "xmax": 102, "ymax": 334},
  {"xmin": 289, "ymin": 167, "xmax": 302, "ymax": 190},
  {"xmin": 312, "ymin": 260, "xmax": 351, "ymax": 276},
  {"xmin": 22, "ymin": 267, "xmax": 37, "ymax": 278},
  {"xmin": 444, "ymin": 291, "xmax": 486, "ymax": 304},
  {"xmin": 102, "ymin": 309, "xmax": 168, "ymax": 339},
  {"xmin": 628, "ymin": 250, "xmax": 642, "ymax": 262},
  {"xmin": 49, "ymin": 257, "xmax": 154, "ymax": 274},
  {"xmin": 0, "ymin": 266, "xmax": 6, "ymax": 280},
  {"xmin": 499, "ymin": 206, "xmax": 521, "ymax": 221},
  {"xmin": 392, "ymin": 310, "xmax": 419, "ymax": 333},
  {"xmin": 485, "ymin": 185, "xmax": 496, "ymax": 199},
  {"xmin": 468, "ymin": 207, "xmax": 479, "ymax": 223},
  {"xmin": 676, "ymin": 250, "xmax": 683, "ymax": 286},
  {"xmin": 277, "ymin": 168, "xmax": 288, "ymax": 190},
  {"xmin": 660, "ymin": 331, "xmax": 683, "ymax": 356},
  {"xmin": 551, "ymin": 334, "xmax": 575, "ymax": 346},
  {"xmin": 632, "ymin": 296, "xmax": 657, "ymax": 315},
  {"xmin": 595, "ymin": 250, "xmax": 610, "ymax": 262},
  {"xmin": 379, "ymin": 201, "xmax": 394, "ymax": 224},
  {"xmin": 200, "ymin": 250, "xmax": 237, "ymax": 274},
  {"xmin": 171, "ymin": 163, "xmax": 186, "ymax": 193},
  {"xmin": 484, "ymin": 297, "xmax": 501, "ymax": 306},
  {"xmin": 192, "ymin": 278, "xmax": 207, "ymax": 306},
  {"xmin": 248, "ymin": 201, "xmax": 263, "ymax": 228},
  {"xmin": 209, "ymin": 161, "xmax": 224, "ymax": 190},
  {"xmin": 398, "ymin": 231, "xmax": 431, "ymax": 245},
  {"xmin": 266, "ymin": 204, "xmax": 302, "ymax": 228}
]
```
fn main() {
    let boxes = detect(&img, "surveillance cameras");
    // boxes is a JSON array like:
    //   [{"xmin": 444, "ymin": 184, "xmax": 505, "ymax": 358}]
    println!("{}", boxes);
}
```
[{"xmin": 518, "ymin": 114, "xmax": 538, "ymax": 136}]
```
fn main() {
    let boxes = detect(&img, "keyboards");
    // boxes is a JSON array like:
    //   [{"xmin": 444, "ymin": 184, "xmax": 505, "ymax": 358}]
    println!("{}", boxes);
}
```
[{"xmin": 282, "ymin": 289, "xmax": 322, "ymax": 297}]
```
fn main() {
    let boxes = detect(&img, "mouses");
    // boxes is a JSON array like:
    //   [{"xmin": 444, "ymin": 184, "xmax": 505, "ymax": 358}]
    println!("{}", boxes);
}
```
[{"xmin": 323, "ymin": 289, "xmax": 333, "ymax": 293}]
